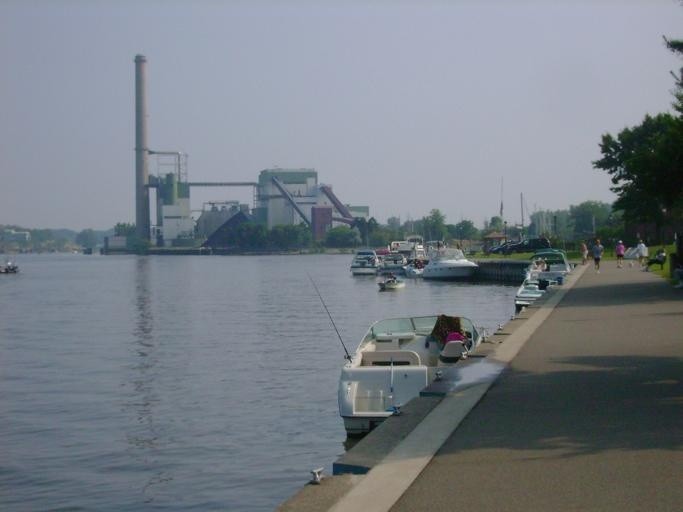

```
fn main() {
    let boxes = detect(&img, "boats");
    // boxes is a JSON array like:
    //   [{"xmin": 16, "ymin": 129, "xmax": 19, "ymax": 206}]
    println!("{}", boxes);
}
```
[
  {"xmin": 377, "ymin": 278, "xmax": 406, "ymax": 289},
  {"xmin": 337, "ymin": 314, "xmax": 483, "ymax": 435},
  {"xmin": 350, "ymin": 240, "xmax": 479, "ymax": 279},
  {"xmin": 514, "ymin": 250, "xmax": 572, "ymax": 307},
  {"xmin": 0, "ymin": 265, "xmax": 19, "ymax": 274}
]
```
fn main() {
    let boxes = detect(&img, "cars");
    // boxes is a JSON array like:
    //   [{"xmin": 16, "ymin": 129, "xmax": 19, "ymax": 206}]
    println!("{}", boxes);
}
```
[{"xmin": 489, "ymin": 241, "xmax": 517, "ymax": 255}]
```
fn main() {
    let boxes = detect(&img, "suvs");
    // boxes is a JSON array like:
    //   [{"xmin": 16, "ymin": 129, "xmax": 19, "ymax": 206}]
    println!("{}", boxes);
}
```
[{"xmin": 511, "ymin": 238, "xmax": 552, "ymax": 253}]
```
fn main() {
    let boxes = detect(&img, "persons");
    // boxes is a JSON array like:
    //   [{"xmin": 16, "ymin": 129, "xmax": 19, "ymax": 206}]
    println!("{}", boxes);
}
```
[
  {"xmin": 616, "ymin": 240, "xmax": 625, "ymax": 268},
  {"xmin": 579, "ymin": 241, "xmax": 588, "ymax": 265},
  {"xmin": 592, "ymin": 240, "xmax": 604, "ymax": 273},
  {"xmin": 638, "ymin": 240, "xmax": 644, "ymax": 267},
  {"xmin": 534, "ymin": 259, "xmax": 546, "ymax": 271},
  {"xmin": 443, "ymin": 324, "xmax": 465, "ymax": 350},
  {"xmin": 641, "ymin": 248, "xmax": 666, "ymax": 272}
]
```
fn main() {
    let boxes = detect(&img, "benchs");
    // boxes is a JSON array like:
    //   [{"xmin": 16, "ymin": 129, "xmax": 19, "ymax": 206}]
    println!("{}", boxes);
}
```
[
  {"xmin": 656, "ymin": 256, "xmax": 667, "ymax": 270},
  {"xmin": 363, "ymin": 351, "xmax": 421, "ymax": 365}
]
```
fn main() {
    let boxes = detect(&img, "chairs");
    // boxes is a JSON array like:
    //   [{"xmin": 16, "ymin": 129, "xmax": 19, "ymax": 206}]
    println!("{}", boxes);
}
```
[{"xmin": 437, "ymin": 341, "xmax": 469, "ymax": 367}]
